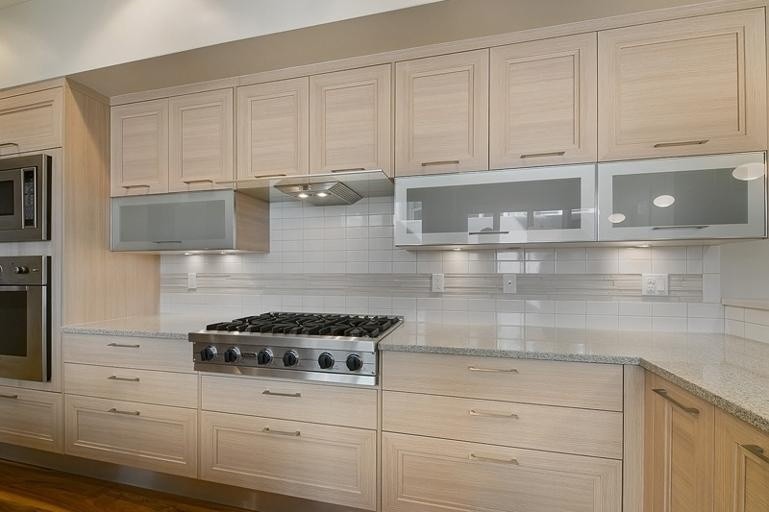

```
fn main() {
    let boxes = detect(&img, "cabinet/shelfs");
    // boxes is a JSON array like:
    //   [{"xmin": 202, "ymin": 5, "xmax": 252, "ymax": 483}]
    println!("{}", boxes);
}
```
[
  {"xmin": 717, "ymin": 401, "xmax": 768, "ymax": 511},
  {"xmin": 109, "ymin": 83, "xmax": 235, "ymax": 193},
  {"xmin": 2, "ymin": 83, "xmax": 63, "ymax": 152},
  {"xmin": 62, "ymin": 333, "xmax": 198, "ymax": 485},
  {"xmin": 1, "ymin": 382, "xmax": 64, "ymax": 457},
  {"xmin": 236, "ymin": 62, "xmax": 395, "ymax": 183},
  {"xmin": 598, "ymin": 6, "xmax": 766, "ymax": 162},
  {"xmin": 644, "ymin": 365, "xmax": 715, "ymax": 509},
  {"xmin": 380, "ymin": 348, "xmax": 631, "ymax": 510},
  {"xmin": 200, "ymin": 372, "xmax": 378, "ymax": 512},
  {"xmin": 396, "ymin": 30, "xmax": 599, "ymax": 169}
]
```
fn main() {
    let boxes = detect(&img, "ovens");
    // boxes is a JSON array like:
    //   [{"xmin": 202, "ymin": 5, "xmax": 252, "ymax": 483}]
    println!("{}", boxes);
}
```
[{"xmin": 0, "ymin": 254, "xmax": 52, "ymax": 383}]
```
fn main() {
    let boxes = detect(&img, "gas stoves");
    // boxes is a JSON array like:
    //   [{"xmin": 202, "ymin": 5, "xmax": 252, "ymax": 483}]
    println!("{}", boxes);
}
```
[{"xmin": 188, "ymin": 310, "xmax": 404, "ymax": 386}]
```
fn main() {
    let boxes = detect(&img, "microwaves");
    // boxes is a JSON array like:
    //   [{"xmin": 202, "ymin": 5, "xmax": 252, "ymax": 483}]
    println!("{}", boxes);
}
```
[{"xmin": 0, "ymin": 153, "xmax": 52, "ymax": 243}]
